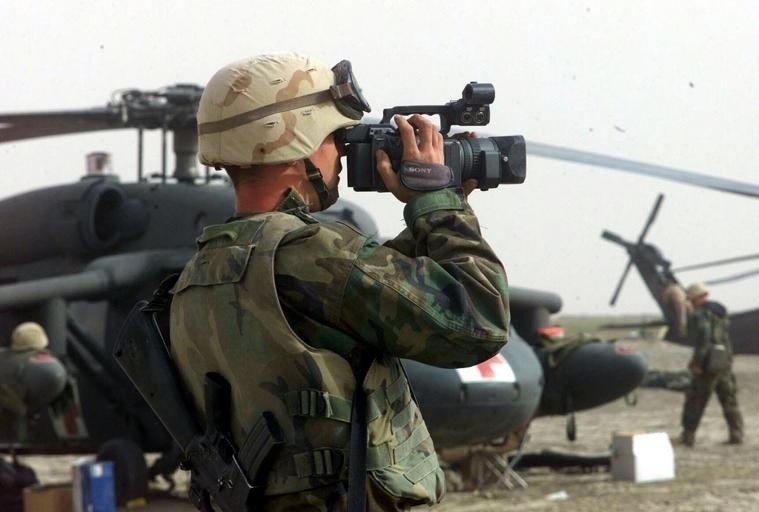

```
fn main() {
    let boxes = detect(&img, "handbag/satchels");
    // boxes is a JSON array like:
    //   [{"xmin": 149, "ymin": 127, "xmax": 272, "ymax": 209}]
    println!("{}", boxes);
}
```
[{"xmin": 705, "ymin": 344, "xmax": 726, "ymax": 373}]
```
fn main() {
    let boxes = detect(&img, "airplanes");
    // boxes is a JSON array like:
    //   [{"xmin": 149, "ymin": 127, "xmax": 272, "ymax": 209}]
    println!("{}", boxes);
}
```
[
  {"xmin": 506, "ymin": 288, "xmax": 651, "ymax": 444},
  {"xmin": 600, "ymin": 192, "xmax": 758, "ymax": 360}
]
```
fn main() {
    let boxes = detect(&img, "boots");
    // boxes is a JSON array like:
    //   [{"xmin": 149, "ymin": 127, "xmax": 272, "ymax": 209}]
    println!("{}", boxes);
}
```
[{"xmin": 670, "ymin": 432, "xmax": 694, "ymax": 447}]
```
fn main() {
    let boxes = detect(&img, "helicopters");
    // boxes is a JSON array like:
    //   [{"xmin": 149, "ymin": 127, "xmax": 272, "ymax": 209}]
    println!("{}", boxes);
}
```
[{"xmin": 0, "ymin": 83, "xmax": 546, "ymax": 501}]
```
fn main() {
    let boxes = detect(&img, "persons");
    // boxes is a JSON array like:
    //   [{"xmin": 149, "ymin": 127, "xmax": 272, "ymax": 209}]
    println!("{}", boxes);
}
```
[
  {"xmin": 672, "ymin": 283, "xmax": 743, "ymax": 446},
  {"xmin": 1, "ymin": 322, "xmax": 75, "ymax": 443},
  {"xmin": 169, "ymin": 53, "xmax": 512, "ymax": 511}
]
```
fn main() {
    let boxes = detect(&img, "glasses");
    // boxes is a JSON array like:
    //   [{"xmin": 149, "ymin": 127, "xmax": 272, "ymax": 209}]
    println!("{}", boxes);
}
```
[{"xmin": 331, "ymin": 58, "xmax": 371, "ymax": 120}]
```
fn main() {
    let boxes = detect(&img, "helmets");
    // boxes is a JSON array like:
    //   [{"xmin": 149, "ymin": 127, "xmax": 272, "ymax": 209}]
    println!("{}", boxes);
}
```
[
  {"xmin": 12, "ymin": 322, "xmax": 48, "ymax": 352},
  {"xmin": 686, "ymin": 285, "xmax": 708, "ymax": 300},
  {"xmin": 196, "ymin": 52, "xmax": 363, "ymax": 167},
  {"xmin": 25, "ymin": 362, "xmax": 66, "ymax": 402}
]
